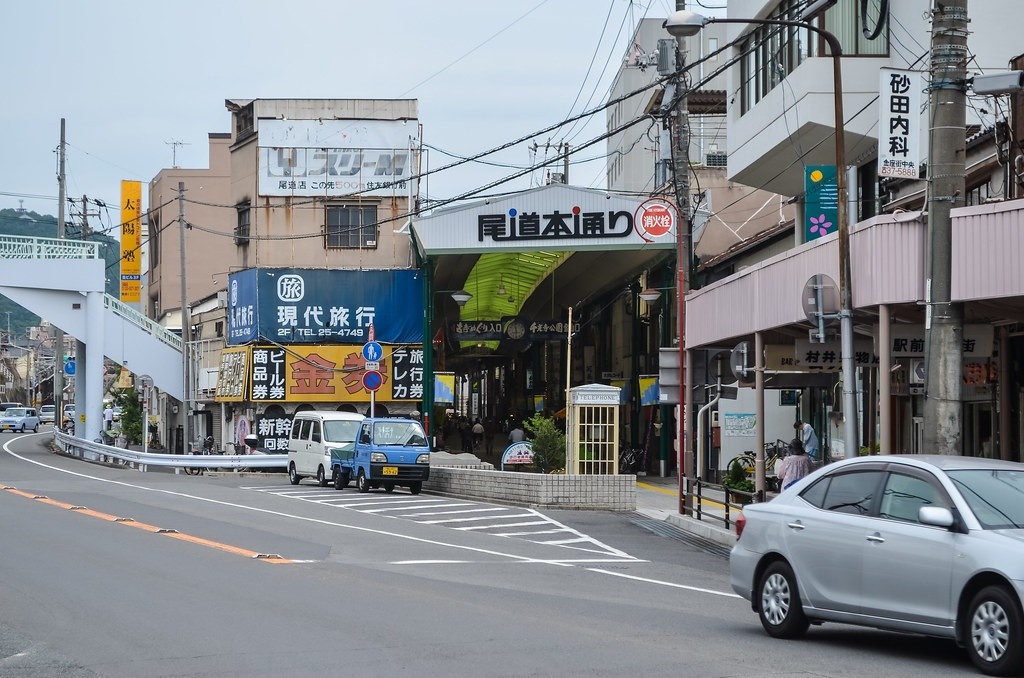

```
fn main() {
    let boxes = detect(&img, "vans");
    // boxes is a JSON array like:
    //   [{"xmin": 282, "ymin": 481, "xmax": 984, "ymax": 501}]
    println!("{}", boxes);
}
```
[{"xmin": 287, "ymin": 411, "xmax": 368, "ymax": 487}]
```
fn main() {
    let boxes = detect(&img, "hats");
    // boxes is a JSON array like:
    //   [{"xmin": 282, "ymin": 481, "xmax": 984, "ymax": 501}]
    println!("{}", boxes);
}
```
[
  {"xmin": 105, "ymin": 404, "xmax": 111, "ymax": 409},
  {"xmin": 787, "ymin": 439, "xmax": 805, "ymax": 455}
]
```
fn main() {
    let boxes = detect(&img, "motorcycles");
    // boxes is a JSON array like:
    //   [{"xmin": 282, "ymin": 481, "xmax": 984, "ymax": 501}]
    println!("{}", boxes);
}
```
[
  {"xmin": 463, "ymin": 437, "xmax": 472, "ymax": 453},
  {"xmin": 63, "ymin": 413, "xmax": 75, "ymax": 455}
]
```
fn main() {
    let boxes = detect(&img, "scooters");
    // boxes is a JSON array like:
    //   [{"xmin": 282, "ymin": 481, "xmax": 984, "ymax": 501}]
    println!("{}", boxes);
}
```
[{"xmin": 244, "ymin": 434, "xmax": 288, "ymax": 473}]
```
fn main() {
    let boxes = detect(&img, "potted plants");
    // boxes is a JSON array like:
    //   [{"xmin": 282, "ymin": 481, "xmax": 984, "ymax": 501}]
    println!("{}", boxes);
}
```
[
  {"xmin": 129, "ymin": 432, "xmax": 143, "ymax": 446},
  {"xmin": 723, "ymin": 461, "xmax": 755, "ymax": 505}
]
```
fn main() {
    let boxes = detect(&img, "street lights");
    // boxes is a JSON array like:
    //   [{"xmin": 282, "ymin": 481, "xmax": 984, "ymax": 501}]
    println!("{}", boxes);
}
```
[
  {"xmin": 7, "ymin": 343, "xmax": 36, "ymax": 408},
  {"xmin": 35, "ymin": 337, "xmax": 57, "ymax": 407},
  {"xmin": 661, "ymin": 11, "xmax": 860, "ymax": 460},
  {"xmin": 5, "ymin": 311, "xmax": 13, "ymax": 342}
]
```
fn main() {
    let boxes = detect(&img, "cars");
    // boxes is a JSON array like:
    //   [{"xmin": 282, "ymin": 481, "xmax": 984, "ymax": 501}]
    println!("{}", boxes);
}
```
[
  {"xmin": 729, "ymin": 454, "xmax": 1024, "ymax": 678},
  {"xmin": 39, "ymin": 405, "xmax": 56, "ymax": 425},
  {"xmin": 0, "ymin": 407, "xmax": 39, "ymax": 433},
  {"xmin": 64, "ymin": 404, "xmax": 75, "ymax": 419},
  {"xmin": 0, "ymin": 402, "xmax": 25, "ymax": 421},
  {"xmin": 113, "ymin": 406, "xmax": 128, "ymax": 422}
]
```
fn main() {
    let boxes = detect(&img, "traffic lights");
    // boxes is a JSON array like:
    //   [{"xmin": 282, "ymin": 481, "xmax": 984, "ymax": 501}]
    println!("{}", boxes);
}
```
[{"xmin": 63, "ymin": 356, "xmax": 75, "ymax": 361}]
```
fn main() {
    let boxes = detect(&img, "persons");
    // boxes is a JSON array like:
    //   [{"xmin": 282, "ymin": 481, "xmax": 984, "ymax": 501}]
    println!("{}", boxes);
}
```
[
  {"xmin": 793, "ymin": 419, "xmax": 820, "ymax": 461},
  {"xmin": 777, "ymin": 438, "xmax": 811, "ymax": 494},
  {"xmin": 445, "ymin": 407, "xmax": 528, "ymax": 457},
  {"xmin": 103, "ymin": 405, "xmax": 115, "ymax": 430}
]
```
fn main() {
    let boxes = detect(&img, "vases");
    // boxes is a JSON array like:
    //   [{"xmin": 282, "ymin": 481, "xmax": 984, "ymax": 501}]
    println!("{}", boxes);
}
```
[
  {"xmin": 139, "ymin": 443, "xmax": 141, "ymax": 446},
  {"xmin": 130, "ymin": 441, "xmax": 134, "ymax": 445}
]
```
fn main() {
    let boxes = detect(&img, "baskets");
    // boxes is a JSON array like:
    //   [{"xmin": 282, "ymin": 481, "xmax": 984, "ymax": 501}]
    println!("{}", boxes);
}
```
[
  {"xmin": 766, "ymin": 447, "xmax": 776, "ymax": 458},
  {"xmin": 234, "ymin": 445, "xmax": 246, "ymax": 454},
  {"xmin": 199, "ymin": 440, "xmax": 212, "ymax": 450}
]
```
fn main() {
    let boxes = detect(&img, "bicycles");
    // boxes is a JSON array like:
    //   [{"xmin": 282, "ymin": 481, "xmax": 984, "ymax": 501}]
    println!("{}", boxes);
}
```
[
  {"xmin": 94, "ymin": 432, "xmax": 128, "ymax": 466},
  {"xmin": 473, "ymin": 435, "xmax": 482, "ymax": 450},
  {"xmin": 205, "ymin": 442, "xmax": 248, "ymax": 472},
  {"xmin": 183, "ymin": 434, "xmax": 219, "ymax": 476}
]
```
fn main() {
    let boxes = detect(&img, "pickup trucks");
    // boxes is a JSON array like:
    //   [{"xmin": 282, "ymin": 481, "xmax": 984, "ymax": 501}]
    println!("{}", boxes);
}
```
[{"xmin": 330, "ymin": 417, "xmax": 431, "ymax": 494}]
